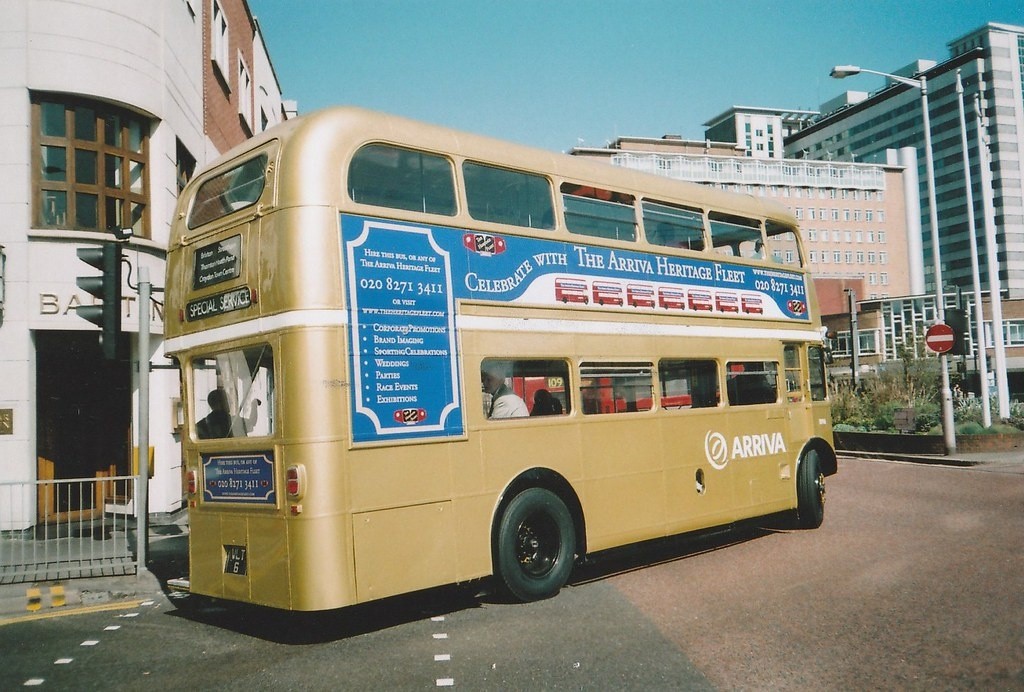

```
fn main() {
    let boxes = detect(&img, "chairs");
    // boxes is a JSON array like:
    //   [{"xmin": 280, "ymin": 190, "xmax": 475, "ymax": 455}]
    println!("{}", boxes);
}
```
[{"xmin": 531, "ymin": 398, "xmax": 563, "ymax": 417}]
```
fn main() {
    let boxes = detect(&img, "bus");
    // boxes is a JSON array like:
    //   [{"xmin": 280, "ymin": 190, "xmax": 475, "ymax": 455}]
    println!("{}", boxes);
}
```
[{"xmin": 161, "ymin": 105, "xmax": 837, "ymax": 615}]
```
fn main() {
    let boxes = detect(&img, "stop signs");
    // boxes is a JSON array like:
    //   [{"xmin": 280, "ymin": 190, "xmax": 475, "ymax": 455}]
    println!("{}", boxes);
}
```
[{"xmin": 924, "ymin": 325, "xmax": 955, "ymax": 354}]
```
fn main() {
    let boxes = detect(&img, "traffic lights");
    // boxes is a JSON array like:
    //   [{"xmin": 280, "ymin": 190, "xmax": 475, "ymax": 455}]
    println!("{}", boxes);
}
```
[{"xmin": 74, "ymin": 239, "xmax": 123, "ymax": 364}]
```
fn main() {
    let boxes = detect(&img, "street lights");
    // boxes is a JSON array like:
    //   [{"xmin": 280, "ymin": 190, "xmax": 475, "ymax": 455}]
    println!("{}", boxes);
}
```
[{"xmin": 827, "ymin": 64, "xmax": 960, "ymax": 459}]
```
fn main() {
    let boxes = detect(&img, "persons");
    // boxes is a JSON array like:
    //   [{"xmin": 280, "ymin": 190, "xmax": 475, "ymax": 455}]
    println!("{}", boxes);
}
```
[
  {"xmin": 482, "ymin": 364, "xmax": 529, "ymax": 418},
  {"xmin": 953, "ymin": 384, "xmax": 963, "ymax": 409},
  {"xmin": 195, "ymin": 389, "xmax": 258, "ymax": 440}
]
